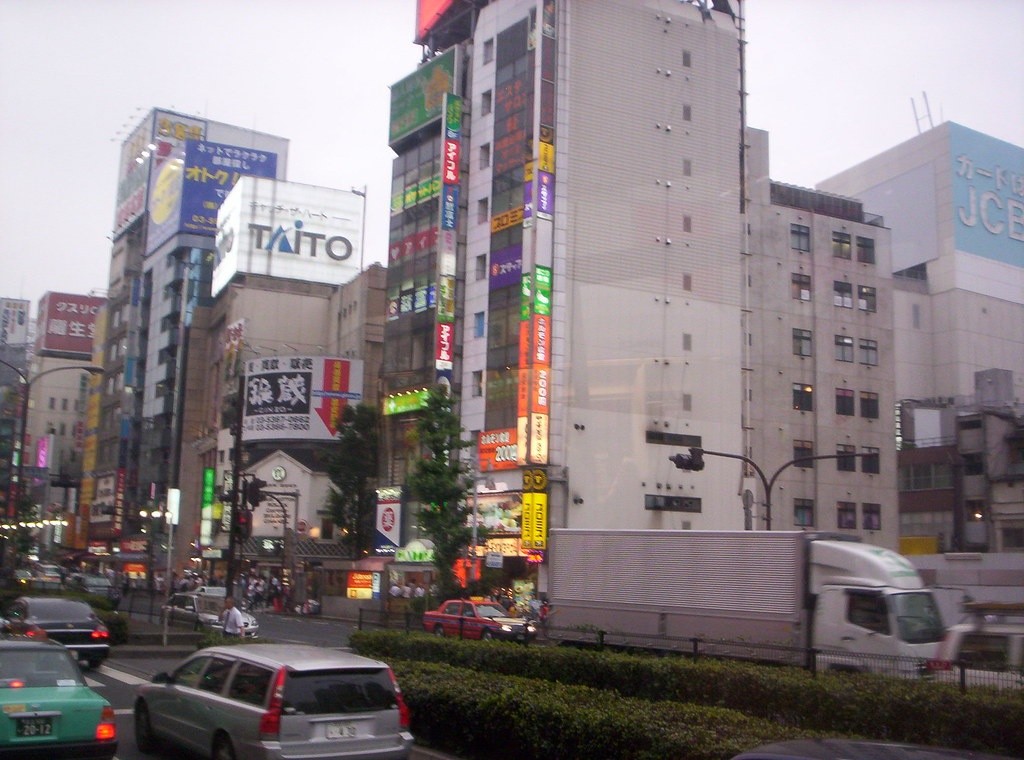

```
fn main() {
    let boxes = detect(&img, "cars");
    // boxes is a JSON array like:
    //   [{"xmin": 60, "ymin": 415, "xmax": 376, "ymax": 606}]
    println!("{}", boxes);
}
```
[
  {"xmin": 70, "ymin": 571, "xmax": 122, "ymax": 607},
  {"xmin": 161, "ymin": 591, "xmax": 260, "ymax": 639},
  {"xmin": 0, "ymin": 629, "xmax": 118, "ymax": 760},
  {"xmin": 422, "ymin": 596, "xmax": 538, "ymax": 645},
  {"xmin": 11, "ymin": 554, "xmax": 93, "ymax": 592},
  {"xmin": 133, "ymin": 642, "xmax": 415, "ymax": 760},
  {"xmin": 0, "ymin": 594, "xmax": 112, "ymax": 669}
]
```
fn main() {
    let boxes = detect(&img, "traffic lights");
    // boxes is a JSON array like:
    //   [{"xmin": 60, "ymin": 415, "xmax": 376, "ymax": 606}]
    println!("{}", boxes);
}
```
[
  {"xmin": 250, "ymin": 479, "xmax": 267, "ymax": 507},
  {"xmin": 235, "ymin": 508, "xmax": 253, "ymax": 539}
]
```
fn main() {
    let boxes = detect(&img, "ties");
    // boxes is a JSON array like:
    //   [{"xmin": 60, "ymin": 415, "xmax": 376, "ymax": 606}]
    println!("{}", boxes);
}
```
[{"xmin": 223, "ymin": 611, "xmax": 230, "ymax": 635}]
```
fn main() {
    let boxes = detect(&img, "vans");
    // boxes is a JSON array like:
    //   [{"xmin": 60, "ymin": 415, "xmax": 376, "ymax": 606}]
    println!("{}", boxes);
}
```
[{"xmin": 941, "ymin": 622, "xmax": 1024, "ymax": 694}]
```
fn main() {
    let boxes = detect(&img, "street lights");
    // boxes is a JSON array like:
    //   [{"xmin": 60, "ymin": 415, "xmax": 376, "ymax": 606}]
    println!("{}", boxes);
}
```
[{"xmin": 0, "ymin": 358, "xmax": 106, "ymax": 514}]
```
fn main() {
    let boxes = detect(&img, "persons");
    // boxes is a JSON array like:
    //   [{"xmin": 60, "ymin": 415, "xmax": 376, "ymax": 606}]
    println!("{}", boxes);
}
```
[
  {"xmin": 172, "ymin": 568, "xmax": 217, "ymax": 593},
  {"xmin": 105, "ymin": 565, "xmax": 167, "ymax": 596},
  {"xmin": 220, "ymin": 597, "xmax": 246, "ymax": 639},
  {"xmin": 241, "ymin": 568, "xmax": 282, "ymax": 610},
  {"xmin": 390, "ymin": 581, "xmax": 441, "ymax": 599}
]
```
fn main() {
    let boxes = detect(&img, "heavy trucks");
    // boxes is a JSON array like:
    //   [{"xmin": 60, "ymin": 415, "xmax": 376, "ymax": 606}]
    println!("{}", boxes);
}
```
[{"xmin": 544, "ymin": 523, "xmax": 945, "ymax": 687}]
